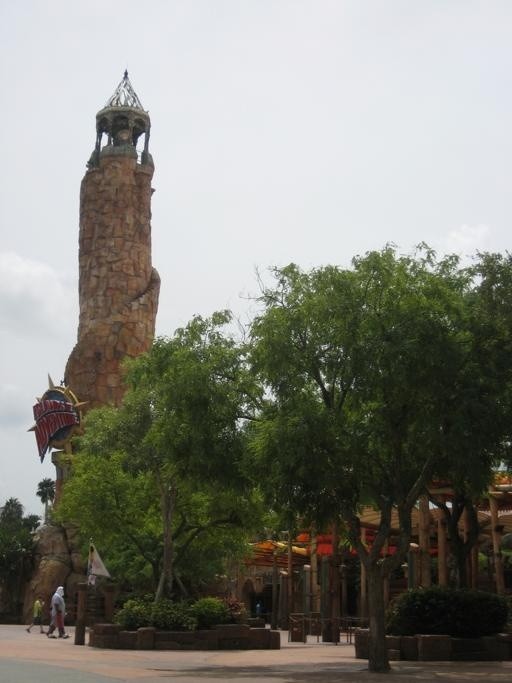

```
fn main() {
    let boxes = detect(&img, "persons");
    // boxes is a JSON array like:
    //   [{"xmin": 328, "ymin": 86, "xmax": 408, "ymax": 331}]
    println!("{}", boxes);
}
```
[
  {"xmin": 26, "ymin": 597, "xmax": 45, "ymax": 635},
  {"xmin": 46, "ymin": 587, "xmax": 69, "ymax": 638}
]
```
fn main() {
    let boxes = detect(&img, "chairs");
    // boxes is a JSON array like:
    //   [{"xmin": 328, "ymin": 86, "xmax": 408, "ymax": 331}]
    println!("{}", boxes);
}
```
[{"xmin": 289, "ymin": 611, "xmax": 367, "ymax": 646}]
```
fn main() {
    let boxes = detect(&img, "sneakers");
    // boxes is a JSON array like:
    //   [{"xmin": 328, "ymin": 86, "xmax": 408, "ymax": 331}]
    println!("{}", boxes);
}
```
[
  {"xmin": 27, "ymin": 629, "xmax": 30, "ymax": 633},
  {"xmin": 40, "ymin": 631, "xmax": 69, "ymax": 639}
]
